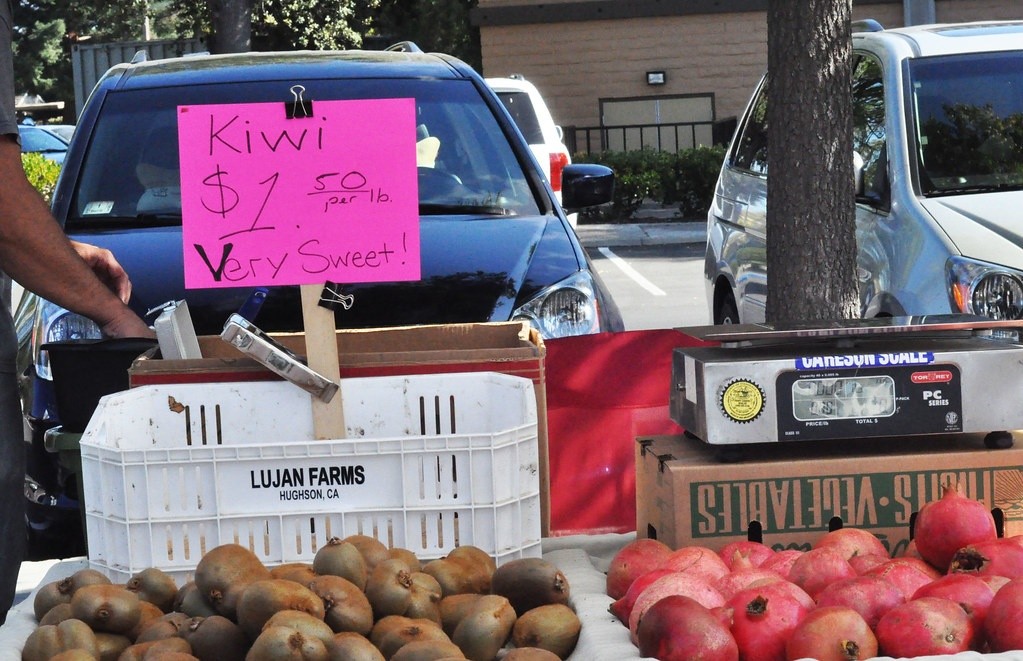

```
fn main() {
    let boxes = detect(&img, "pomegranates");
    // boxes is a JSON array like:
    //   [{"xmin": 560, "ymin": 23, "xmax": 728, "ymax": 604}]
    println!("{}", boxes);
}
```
[{"xmin": 606, "ymin": 479, "xmax": 1023, "ymax": 661}]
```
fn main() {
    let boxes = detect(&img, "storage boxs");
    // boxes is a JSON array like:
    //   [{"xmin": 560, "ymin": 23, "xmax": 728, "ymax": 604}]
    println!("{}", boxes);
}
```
[
  {"xmin": 78, "ymin": 381, "xmax": 542, "ymax": 590},
  {"xmin": 128, "ymin": 319, "xmax": 550, "ymax": 537},
  {"xmin": 634, "ymin": 434, "xmax": 1023, "ymax": 558},
  {"xmin": 38, "ymin": 338, "xmax": 158, "ymax": 434}
]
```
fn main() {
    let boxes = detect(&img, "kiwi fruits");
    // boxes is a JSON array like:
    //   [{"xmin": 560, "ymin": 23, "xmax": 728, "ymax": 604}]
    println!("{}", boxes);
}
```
[{"xmin": 21, "ymin": 534, "xmax": 581, "ymax": 661}]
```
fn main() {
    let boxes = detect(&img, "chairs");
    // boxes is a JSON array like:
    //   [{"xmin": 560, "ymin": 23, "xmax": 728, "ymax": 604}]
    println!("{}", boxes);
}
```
[
  {"xmin": 919, "ymin": 95, "xmax": 981, "ymax": 177},
  {"xmin": 132, "ymin": 126, "xmax": 179, "ymax": 199},
  {"xmin": 363, "ymin": 84, "xmax": 446, "ymax": 169}
]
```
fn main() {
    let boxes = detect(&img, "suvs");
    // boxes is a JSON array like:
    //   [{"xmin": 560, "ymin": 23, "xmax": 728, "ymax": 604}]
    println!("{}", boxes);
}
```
[
  {"xmin": 693, "ymin": 16, "xmax": 1023, "ymax": 348},
  {"xmin": 13, "ymin": 39, "xmax": 627, "ymax": 564},
  {"xmin": 482, "ymin": 71, "xmax": 581, "ymax": 237}
]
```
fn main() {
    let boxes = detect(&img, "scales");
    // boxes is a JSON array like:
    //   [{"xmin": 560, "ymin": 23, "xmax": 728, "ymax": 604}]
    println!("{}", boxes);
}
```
[{"xmin": 667, "ymin": 314, "xmax": 1023, "ymax": 464}]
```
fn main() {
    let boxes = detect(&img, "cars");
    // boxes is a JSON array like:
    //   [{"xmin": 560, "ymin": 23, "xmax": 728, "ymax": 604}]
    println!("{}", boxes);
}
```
[{"xmin": 16, "ymin": 123, "xmax": 78, "ymax": 169}]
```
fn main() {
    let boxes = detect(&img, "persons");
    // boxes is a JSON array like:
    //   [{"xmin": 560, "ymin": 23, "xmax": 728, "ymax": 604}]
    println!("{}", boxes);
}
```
[{"xmin": 0, "ymin": 0, "xmax": 155, "ymax": 623}]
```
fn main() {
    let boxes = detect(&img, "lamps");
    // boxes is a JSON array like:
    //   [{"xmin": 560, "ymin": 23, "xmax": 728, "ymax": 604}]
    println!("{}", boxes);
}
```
[{"xmin": 645, "ymin": 70, "xmax": 666, "ymax": 86}]
